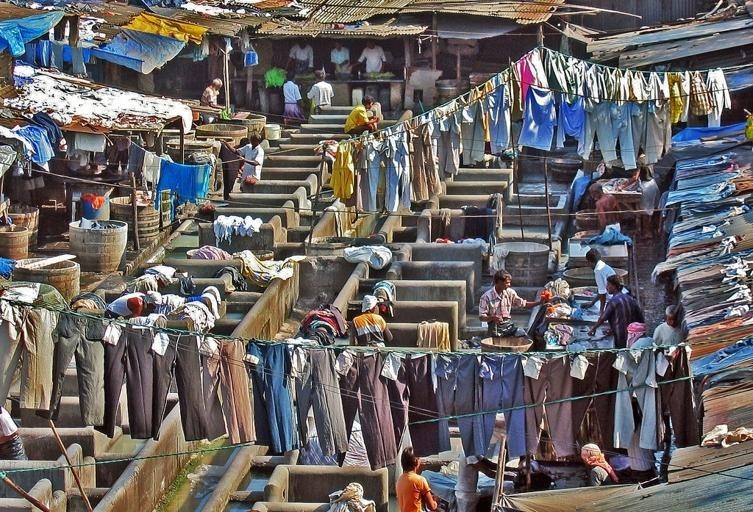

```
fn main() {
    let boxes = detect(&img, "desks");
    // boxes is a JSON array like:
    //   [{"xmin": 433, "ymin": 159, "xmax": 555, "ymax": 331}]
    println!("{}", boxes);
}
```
[{"xmin": 594, "ymin": 179, "xmax": 643, "ymax": 238}]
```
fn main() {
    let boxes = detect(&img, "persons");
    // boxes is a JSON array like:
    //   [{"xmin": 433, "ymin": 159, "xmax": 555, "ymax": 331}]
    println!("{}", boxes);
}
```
[
  {"xmin": 617, "ymin": 158, "xmax": 662, "ymax": 240},
  {"xmin": 588, "ymin": 182, "xmax": 625, "ymax": 235},
  {"xmin": 200, "ymin": 38, "xmax": 387, "ymax": 135},
  {"xmin": 104, "ymin": 290, "xmax": 163, "ymax": 318},
  {"xmin": 455, "ymin": 447, "xmax": 520, "ymax": 512},
  {"xmin": 394, "ymin": 445, "xmax": 439, "ymax": 512},
  {"xmin": 349, "ymin": 294, "xmax": 394, "ymax": 348},
  {"xmin": 220, "ymin": 133, "xmax": 266, "ymax": 181},
  {"xmin": 580, "ymin": 248, "xmax": 686, "ymax": 349},
  {"xmin": 479, "ymin": 267, "xmax": 546, "ymax": 337},
  {"xmin": 0, "ymin": 406, "xmax": 29, "ymax": 460},
  {"xmin": 120, "ymin": 272, "xmax": 173, "ymax": 292},
  {"xmin": 578, "ymin": 442, "xmax": 618, "ymax": 486}
]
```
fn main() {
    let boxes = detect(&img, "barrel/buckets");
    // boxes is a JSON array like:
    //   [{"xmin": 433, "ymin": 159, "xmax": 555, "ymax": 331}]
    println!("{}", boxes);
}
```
[
  {"xmin": 562, "ymin": 266, "xmax": 630, "ymax": 288},
  {"xmin": 167, "ymin": 113, "xmax": 280, "ymax": 191},
  {"xmin": 109, "ymin": 195, "xmax": 161, "ymax": 244},
  {"xmin": 69, "ymin": 220, "xmax": 129, "ymax": 273},
  {"xmin": 493, "ymin": 241, "xmax": 550, "ymax": 315},
  {"xmin": 0, "ymin": 226, "xmax": 28, "ymax": 259},
  {"xmin": 550, "ymin": 158, "xmax": 583, "ymax": 181},
  {"xmin": 13, "ymin": 258, "xmax": 80, "ymax": 304},
  {"xmin": 6, "ymin": 205, "xmax": 40, "ymax": 247},
  {"xmin": 482, "ymin": 337, "xmax": 533, "ymax": 353},
  {"xmin": 575, "ymin": 208, "xmax": 600, "ymax": 230},
  {"xmin": 434, "ymin": 79, "xmax": 470, "ymax": 105}
]
